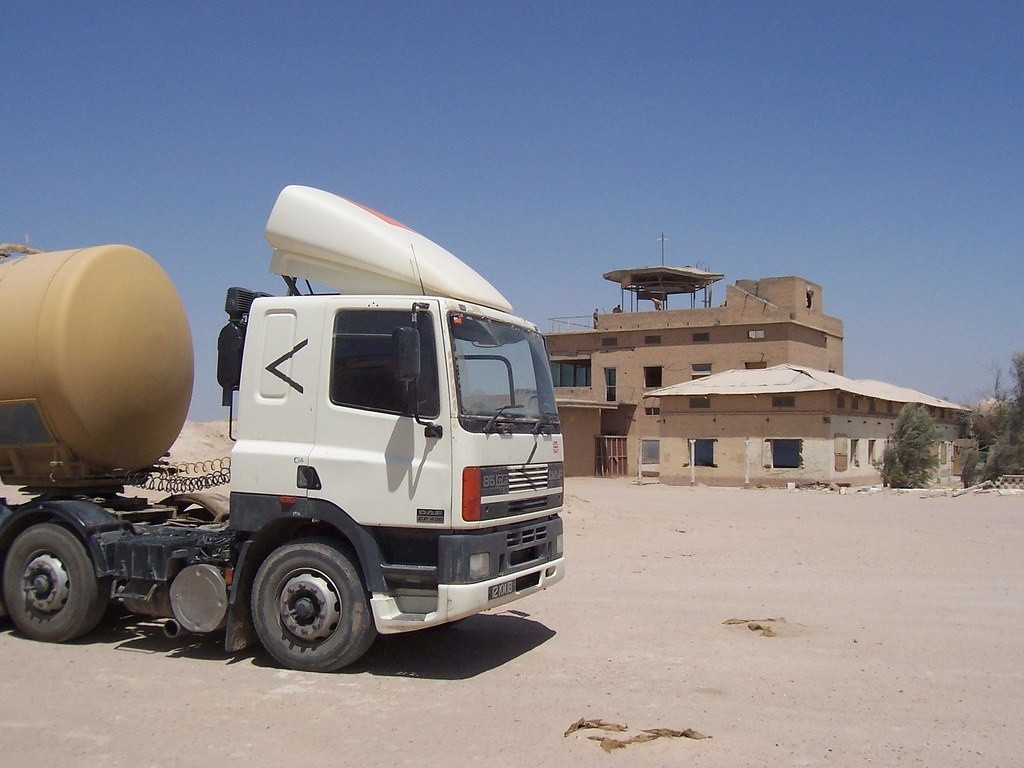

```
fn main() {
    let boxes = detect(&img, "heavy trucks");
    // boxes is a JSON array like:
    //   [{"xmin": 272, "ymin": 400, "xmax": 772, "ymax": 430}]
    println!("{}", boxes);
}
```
[{"xmin": 0, "ymin": 184, "xmax": 566, "ymax": 673}]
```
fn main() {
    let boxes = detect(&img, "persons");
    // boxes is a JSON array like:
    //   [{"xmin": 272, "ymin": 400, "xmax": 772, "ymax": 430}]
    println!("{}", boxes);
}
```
[
  {"xmin": 593, "ymin": 309, "xmax": 598, "ymax": 329},
  {"xmin": 652, "ymin": 298, "xmax": 662, "ymax": 311},
  {"xmin": 612, "ymin": 305, "xmax": 621, "ymax": 313}
]
